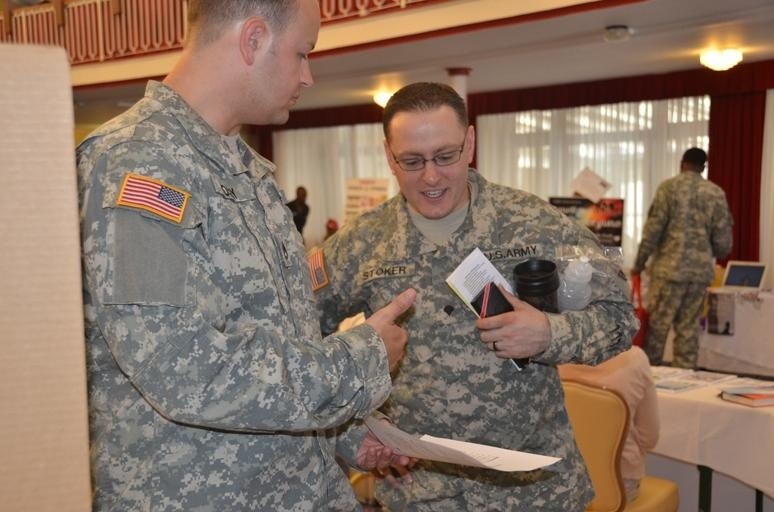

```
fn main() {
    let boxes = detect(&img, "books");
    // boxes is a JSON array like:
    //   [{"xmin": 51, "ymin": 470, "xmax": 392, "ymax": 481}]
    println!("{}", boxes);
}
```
[
  {"xmin": 470, "ymin": 281, "xmax": 528, "ymax": 368},
  {"xmin": 655, "ymin": 369, "xmax": 737, "ymax": 393},
  {"xmin": 445, "ymin": 246, "xmax": 523, "ymax": 372},
  {"xmin": 721, "ymin": 390, "xmax": 774, "ymax": 408},
  {"xmin": 720, "ymin": 381, "xmax": 774, "ymax": 394}
]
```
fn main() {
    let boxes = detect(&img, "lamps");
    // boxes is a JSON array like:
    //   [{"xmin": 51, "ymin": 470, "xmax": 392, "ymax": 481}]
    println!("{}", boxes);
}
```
[
  {"xmin": 699, "ymin": 47, "xmax": 743, "ymax": 71},
  {"xmin": 603, "ymin": 24, "xmax": 630, "ymax": 43}
]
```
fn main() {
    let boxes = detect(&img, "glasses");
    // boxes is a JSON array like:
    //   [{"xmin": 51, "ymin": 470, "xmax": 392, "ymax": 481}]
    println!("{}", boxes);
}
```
[{"xmin": 388, "ymin": 134, "xmax": 466, "ymax": 171}]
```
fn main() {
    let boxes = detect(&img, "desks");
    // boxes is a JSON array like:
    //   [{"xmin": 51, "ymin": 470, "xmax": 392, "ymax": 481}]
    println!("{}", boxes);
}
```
[{"xmin": 647, "ymin": 365, "xmax": 774, "ymax": 512}]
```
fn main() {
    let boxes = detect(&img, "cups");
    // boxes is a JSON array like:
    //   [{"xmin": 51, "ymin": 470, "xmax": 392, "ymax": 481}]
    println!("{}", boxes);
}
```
[{"xmin": 512, "ymin": 260, "xmax": 560, "ymax": 314}]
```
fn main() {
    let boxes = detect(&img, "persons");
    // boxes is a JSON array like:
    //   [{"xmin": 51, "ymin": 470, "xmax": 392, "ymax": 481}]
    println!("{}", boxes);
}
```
[
  {"xmin": 77, "ymin": 1, "xmax": 416, "ymax": 510},
  {"xmin": 304, "ymin": 83, "xmax": 640, "ymax": 511},
  {"xmin": 721, "ymin": 322, "xmax": 731, "ymax": 334},
  {"xmin": 553, "ymin": 344, "xmax": 659, "ymax": 501},
  {"xmin": 707, "ymin": 293, "xmax": 718, "ymax": 334},
  {"xmin": 631, "ymin": 147, "xmax": 734, "ymax": 368},
  {"xmin": 284, "ymin": 186, "xmax": 308, "ymax": 233}
]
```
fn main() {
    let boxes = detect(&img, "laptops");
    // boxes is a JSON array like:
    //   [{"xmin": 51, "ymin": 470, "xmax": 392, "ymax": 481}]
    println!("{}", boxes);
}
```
[{"xmin": 706, "ymin": 260, "xmax": 768, "ymax": 294}]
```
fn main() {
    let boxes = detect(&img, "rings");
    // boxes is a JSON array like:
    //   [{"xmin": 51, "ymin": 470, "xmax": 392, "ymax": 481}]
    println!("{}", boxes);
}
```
[{"xmin": 492, "ymin": 341, "xmax": 498, "ymax": 352}]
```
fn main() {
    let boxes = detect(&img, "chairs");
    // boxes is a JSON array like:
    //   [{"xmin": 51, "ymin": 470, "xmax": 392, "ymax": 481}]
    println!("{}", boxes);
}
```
[{"xmin": 559, "ymin": 378, "xmax": 679, "ymax": 512}]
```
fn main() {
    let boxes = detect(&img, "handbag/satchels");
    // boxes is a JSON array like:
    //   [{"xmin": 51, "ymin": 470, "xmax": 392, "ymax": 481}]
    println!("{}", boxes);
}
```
[{"xmin": 630, "ymin": 276, "xmax": 647, "ymax": 345}]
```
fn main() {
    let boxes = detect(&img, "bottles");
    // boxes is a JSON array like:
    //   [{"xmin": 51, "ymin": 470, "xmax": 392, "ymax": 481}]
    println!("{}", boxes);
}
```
[{"xmin": 556, "ymin": 255, "xmax": 593, "ymax": 313}]
